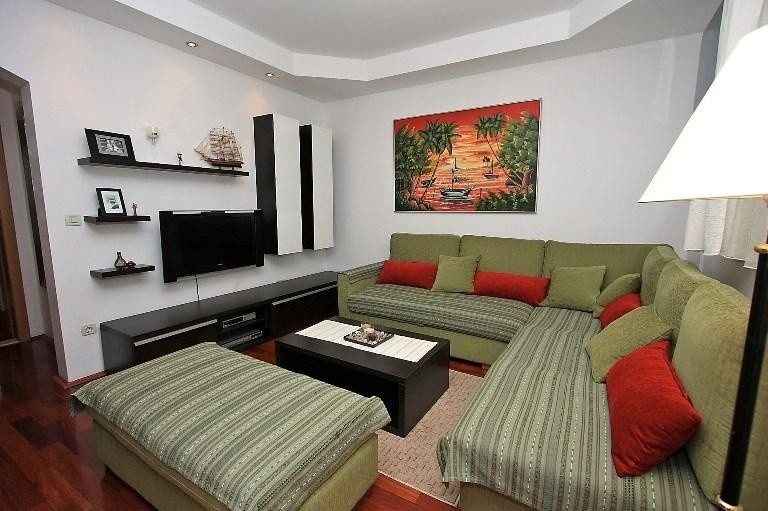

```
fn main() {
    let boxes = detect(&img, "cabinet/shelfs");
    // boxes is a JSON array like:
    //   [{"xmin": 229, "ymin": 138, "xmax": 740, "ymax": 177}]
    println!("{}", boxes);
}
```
[{"xmin": 75, "ymin": 156, "xmax": 250, "ymax": 277}]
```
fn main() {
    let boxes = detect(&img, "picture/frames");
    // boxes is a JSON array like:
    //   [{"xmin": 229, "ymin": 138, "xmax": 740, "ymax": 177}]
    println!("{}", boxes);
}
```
[
  {"xmin": 83, "ymin": 127, "xmax": 137, "ymax": 161},
  {"xmin": 95, "ymin": 188, "xmax": 128, "ymax": 218},
  {"xmin": 393, "ymin": 97, "xmax": 543, "ymax": 215}
]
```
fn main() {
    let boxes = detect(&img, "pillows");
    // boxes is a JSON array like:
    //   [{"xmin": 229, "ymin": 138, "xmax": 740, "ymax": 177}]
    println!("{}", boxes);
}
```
[
  {"xmin": 430, "ymin": 254, "xmax": 482, "ymax": 293},
  {"xmin": 605, "ymin": 340, "xmax": 702, "ymax": 478},
  {"xmin": 537, "ymin": 264, "xmax": 608, "ymax": 313},
  {"xmin": 464, "ymin": 270, "xmax": 551, "ymax": 308},
  {"xmin": 598, "ymin": 294, "xmax": 642, "ymax": 329},
  {"xmin": 591, "ymin": 272, "xmax": 642, "ymax": 319},
  {"xmin": 584, "ymin": 303, "xmax": 674, "ymax": 385},
  {"xmin": 375, "ymin": 256, "xmax": 439, "ymax": 290}
]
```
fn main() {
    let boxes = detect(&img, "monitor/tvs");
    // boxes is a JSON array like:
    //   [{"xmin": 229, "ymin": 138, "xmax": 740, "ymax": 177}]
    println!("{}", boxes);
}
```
[{"xmin": 158, "ymin": 209, "xmax": 264, "ymax": 284}]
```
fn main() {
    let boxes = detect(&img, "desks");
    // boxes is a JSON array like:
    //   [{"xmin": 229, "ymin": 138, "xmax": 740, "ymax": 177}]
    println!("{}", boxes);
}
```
[
  {"xmin": 274, "ymin": 315, "xmax": 451, "ymax": 440},
  {"xmin": 99, "ymin": 270, "xmax": 339, "ymax": 377}
]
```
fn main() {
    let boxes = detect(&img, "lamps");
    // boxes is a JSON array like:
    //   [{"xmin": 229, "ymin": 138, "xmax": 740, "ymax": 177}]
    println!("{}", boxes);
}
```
[
  {"xmin": 147, "ymin": 125, "xmax": 160, "ymax": 145},
  {"xmin": 635, "ymin": 21, "xmax": 768, "ymax": 510}
]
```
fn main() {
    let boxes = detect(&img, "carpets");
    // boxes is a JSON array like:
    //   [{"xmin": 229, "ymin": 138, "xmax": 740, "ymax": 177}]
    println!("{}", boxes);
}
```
[{"xmin": 378, "ymin": 369, "xmax": 485, "ymax": 510}]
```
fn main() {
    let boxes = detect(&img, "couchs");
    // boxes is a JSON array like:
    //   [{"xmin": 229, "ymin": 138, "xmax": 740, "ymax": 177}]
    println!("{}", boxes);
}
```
[
  {"xmin": 66, "ymin": 341, "xmax": 392, "ymax": 511},
  {"xmin": 336, "ymin": 232, "xmax": 676, "ymax": 373},
  {"xmin": 436, "ymin": 246, "xmax": 768, "ymax": 511}
]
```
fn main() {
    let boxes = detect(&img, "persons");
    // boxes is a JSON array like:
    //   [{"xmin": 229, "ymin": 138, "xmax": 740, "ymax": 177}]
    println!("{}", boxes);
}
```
[
  {"xmin": 106, "ymin": 139, "xmax": 110, "ymax": 151},
  {"xmin": 106, "ymin": 138, "xmax": 122, "ymax": 153}
]
